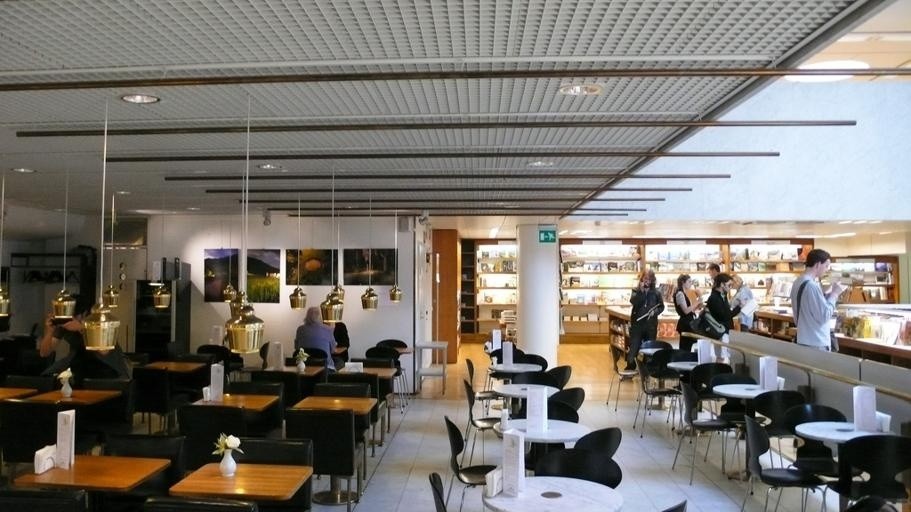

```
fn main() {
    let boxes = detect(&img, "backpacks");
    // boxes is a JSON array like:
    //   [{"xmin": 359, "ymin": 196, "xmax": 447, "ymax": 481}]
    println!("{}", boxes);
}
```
[{"xmin": 689, "ymin": 307, "xmax": 725, "ymax": 340}]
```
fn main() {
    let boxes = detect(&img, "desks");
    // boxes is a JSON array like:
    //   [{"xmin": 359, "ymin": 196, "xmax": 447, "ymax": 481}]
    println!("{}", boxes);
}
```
[
  {"xmin": 292, "ymin": 397, "xmax": 378, "ymax": 505},
  {"xmin": 795, "ymin": 422, "xmax": 895, "ymax": 511},
  {"xmin": 0, "ymin": 387, "xmax": 38, "ymax": 400},
  {"xmin": 169, "ymin": 463, "xmax": 314, "ymax": 499},
  {"xmin": 490, "ymin": 364, "xmax": 542, "ymax": 385},
  {"xmin": 338, "ymin": 367, "xmax": 397, "ymax": 379},
  {"xmin": 494, "ymin": 419, "xmax": 590, "ymax": 444},
  {"xmin": 604, "ymin": 305, "xmax": 741, "ymax": 373},
  {"xmin": 146, "ymin": 361, "xmax": 207, "ymax": 436},
  {"xmin": 14, "ymin": 455, "xmax": 171, "ymax": 492},
  {"xmin": 639, "ymin": 349, "xmax": 669, "ymax": 410},
  {"xmin": 713, "ymin": 385, "xmax": 765, "ymax": 481},
  {"xmin": 668, "ymin": 362, "xmax": 701, "ymax": 437},
  {"xmin": 492, "ymin": 384, "xmax": 560, "ymax": 418},
  {"xmin": 482, "ymin": 476, "xmax": 624, "ymax": 512},
  {"xmin": 26, "ymin": 389, "xmax": 122, "ymax": 405},
  {"xmin": 191, "ymin": 393, "xmax": 279, "ymax": 415},
  {"xmin": 265, "ymin": 365, "xmax": 325, "ymax": 377}
]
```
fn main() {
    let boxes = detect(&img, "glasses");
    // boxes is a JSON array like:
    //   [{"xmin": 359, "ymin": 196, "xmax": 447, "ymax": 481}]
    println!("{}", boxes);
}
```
[{"xmin": 715, "ymin": 283, "xmax": 735, "ymax": 289}]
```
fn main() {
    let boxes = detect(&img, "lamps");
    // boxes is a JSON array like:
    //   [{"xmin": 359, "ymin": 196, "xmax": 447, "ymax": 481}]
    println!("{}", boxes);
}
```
[
  {"xmin": 289, "ymin": 195, "xmax": 307, "ymax": 308},
  {"xmin": 362, "ymin": 198, "xmax": 378, "ymax": 310},
  {"xmin": 229, "ymin": 169, "xmax": 250, "ymax": 319},
  {"xmin": 226, "ymin": 94, "xmax": 264, "ymax": 354},
  {"xmin": 390, "ymin": 209, "xmax": 402, "ymax": 304},
  {"xmin": 52, "ymin": 173, "xmax": 76, "ymax": 319},
  {"xmin": 0, "ymin": 174, "xmax": 9, "ymax": 318},
  {"xmin": 103, "ymin": 189, "xmax": 119, "ymax": 308},
  {"xmin": 82, "ymin": 95, "xmax": 119, "ymax": 351},
  {"xmin": 320, "ymin": 172, "xmax": 344, "ymax": 325},
  {"xmin": 331, "ymin": 211, "xmax": 344, "ymax": 301},
  {"xmin": 153, "ymin": 210, "xmax": 171, "ymax": 308},
  {"xmin": 223, "ymin": 208, "xmax": 237, "ymax": 301}
]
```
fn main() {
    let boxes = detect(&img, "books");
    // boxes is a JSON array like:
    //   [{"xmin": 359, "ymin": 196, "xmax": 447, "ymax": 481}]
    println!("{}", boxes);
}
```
[{"xmin": 740, "ymin": 299, "xmax": 759, "ymax": 316}]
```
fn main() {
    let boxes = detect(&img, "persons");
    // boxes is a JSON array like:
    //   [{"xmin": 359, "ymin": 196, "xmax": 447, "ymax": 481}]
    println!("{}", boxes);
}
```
[
  {"xmin": 40, "ymin": 294, "xmax": 130, "ymax": 380},
  {"xmin": 625, "ymin": 269, "xmax": 664, "ymax": 371},
  {"xmin": 707, "ymin": 274, "xmax": 743, "ymax": 330},
  {"xmin": 673, "ymin": 274, "xmax": 703, "ymax": 353},
  {"xmin": 729, "ymin": 274, "xmax": 753, "ymax": 332},
  {"xmin": 790, "ymin": 249, "xmax": 843, "ymax": 353},
  {"xmin": 294, "ymin": 307, "xmax": 336, "ymax": 374},
  {"xmin": 706, "ymin": 264, "xmax": 720, "ymax": 280},
  {"xmin": 330, "ymin": 321, "xmax": 349, "ymax": 370}
]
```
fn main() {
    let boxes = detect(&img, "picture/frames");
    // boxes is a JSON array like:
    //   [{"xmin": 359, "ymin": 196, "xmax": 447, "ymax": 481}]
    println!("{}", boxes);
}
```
[
  {"xmin": 343, "ymin": 249, "xmax": 398, "ymax": 285},
  {"xmin": 286, "ymin": 248, "xmax": 338, "ymax": 286},
  {"xmin": 205, "ymin": 248, "xmax": 280, "ymax": 303}
]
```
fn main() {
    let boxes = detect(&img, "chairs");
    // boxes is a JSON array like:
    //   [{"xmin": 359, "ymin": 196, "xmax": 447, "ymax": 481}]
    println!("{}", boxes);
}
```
[
  {"xmin": 351, "ymin": 359, "xmax": 394, "ymax": 442},
  {"xmin": 785, "ymin": 405, "xmax": 846, "ymax": 512},
  {"xmin": 520, "ymin": 401, "xmax": 579, "ymax": 470},
  {"xmin": 315, "ymin": 383, "xmax": 371, "ymax": 480},
  {"xmin": 466, "ymin": 359, "xmax": 498, "ymax": 415},
  {"xmin": 292, "ymin": 348, "xmax": 327, "ymax": 359},
  {"xmin": 262, "ymin": 371, "xmax": 301, "ymax": 420},
  {"xmin": 130, "ymin": 368, "xmax": 173, "ymax": 435},
  {"xmin": 232, "ymin": 438, "xmax": 313, "ymax": 512},
  {"xmin": 3, "ymin": 401, "xmax": 113, "ymax": 479},
  {"xmin": 284, "ymin": 409, "xmax": 366, "ymax": 511},
  {"xmin": 608, "ymin": 343, "xmax": 640, "ymax": 412},
  {"xmin": 429, "ymin": 472, "xmax": 447, "ymax": 512},
  {"xmin": 711, "ymin": 374, "xmax": 757, "ymax": 465},
  {"xmin": 285, "ymin": 358, "xmax": 327, "ymax": 384},
  {"xmin": 485, "ymin": 348, "xmax": 524, "ymax": 390},
  {"xmin": 669, "ymin": 349, "xmax": 698, "ymax": 433},
  {"xmin": 825, "ymin": 435, "xmax": 910, "ymax": 512},
  {"xmin": 376, "ymin": 340, "xmax": 410, "ymax": 405},
  {"xmin": 461, "ymin": 379, "xmax": 501, "ymax": 467},
  {"xmin": 178, "ymin": 404, "xmax": 247, "ymax": 472},
  {"xmin": 662, "ymin": 500, "xmax": 689, "ymax": 512},
  {"xmin": 109, "ymin": 434, "xmax": 184, "ymax": 497},
  {"xmin": 366, "ymin": 347, "xmax": 404, "ymax": 414},
  {"xmin": 742, "ymin": 415, "xmax": 823, "ymax": 512},
  {"xmin": 445, "ymin": 415, "xmax": 497, "ymax": 512},
  {"xmin": 547, "ymin": 388, "xmax": 585, "ymax": 411},
  {"xmin": 544, "ymin": 366, "xmax": 571, "ymax": 389},
  {"xmin": 265, "ymin": 365, "xmax": 325, "ymax": 396},
  {"xmin": 633, "ymin": 347, "xmax": 675, "ymax": 437},
  {"xmin": 514, "ymin": 355, "xmax": 547, "ymax": 373},
  {"xmin": 229, "ymin": 382, "xmax": 286, "ymax": 440},
  {"xmin": 513, "ymin": 374, "xmax": 562, "ymax": 418},
  {"xmin": 197, "ymin": 344, "xmax": 241, "ymax": 384},
  {"xmin": 641, "ymin": 340, "xmax": 673, "ymax": 416},
  {"xmin": 574, "ymin": 428, "xmax": 622, "ymax": 458},
  {"xmin": 748, "ymin": 390, "xmax": 805, "ymax": 471},
  {"xmin": 0, "ymin": 487, "xmax": 87, "ymax": 511},
  {"xmin": 145, "ymin": 496, "xmax": 258, "ymax": 511},
  {"xmin": 693, "ymin": 362, "xmax": 733, "ymax": 399},
  {"xmin": 182, "ymin": 353, "xmax": 214, "ymax": 391},
  {"xmin": 672, "ymin": 378, "xmax": 741, "ymax": 486},
  {"xmin": 533, "ymin": 449, "xmax": 622, "ymax": 489},
  {"xmin": 327, "ymin": 373, "xmax": 386, "ymax": 457}
]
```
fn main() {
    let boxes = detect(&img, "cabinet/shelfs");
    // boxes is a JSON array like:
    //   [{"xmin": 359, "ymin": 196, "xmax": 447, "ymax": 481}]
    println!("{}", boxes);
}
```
[
  {"xmin": 416, "ymin": 341, "xmax": 448, "ymax": 395},
  {"xmin": 136, "ymin": 279, "xmax": 191, "ymax": 361},
  {"xmin": 817, "ymin": 254, "xmax": 908, "ymax": 305},
  {"xmin": 432, "ymin": 229, "xmax": 462, "ymax": 364},
  {"xmin": 730, "ymin": 240, "xmax": 814, "ymax": 307},
  {"xmin": 754, "ymin": 302, "xmax": 911, "ymax": 367},
  {"xmin": 559, "ymin": 239, "xmax": 642, "ymax": 345},
  {"xmin": 460, "ymin": 238, "xmax": 476, "ymax": 343},
  {"xmin": 642, "ymin": 239, "xmax": 730, "ymax": 340},
  {"xmin": 476, "ymin": 238, "xmax": 519, "ymax": 344}
]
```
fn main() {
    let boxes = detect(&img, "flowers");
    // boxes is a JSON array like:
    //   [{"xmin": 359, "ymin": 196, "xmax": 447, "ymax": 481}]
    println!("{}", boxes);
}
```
[
  {"xmin": 295, "ymin": 348, "xmax": 310, "ymax": 362},
  {"xmin": 213, "ymin": 432, "xmax": 243, "ymax": 456},
  {"xmin": 56, "ymin": 368, "xmax": 73, "ymax": 382}
]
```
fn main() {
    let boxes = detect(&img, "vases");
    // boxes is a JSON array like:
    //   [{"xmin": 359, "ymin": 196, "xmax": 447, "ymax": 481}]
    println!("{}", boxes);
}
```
[
  {"xmin": 298, "ymin": 361, "xmax": 306, "ymax": 372},
  {"xmin": 220, "ymin": 449, "xmax": 237, "ymax": 477},
  {"xmin": 62, "ymin": 382, "xmax": 72, "ymax": 397}
]
